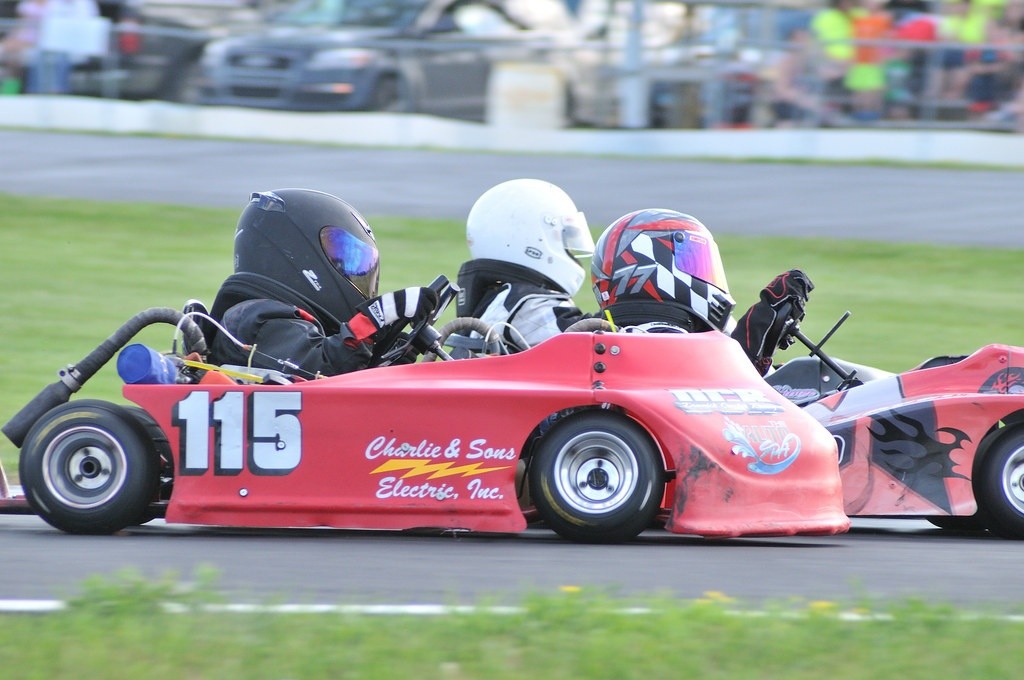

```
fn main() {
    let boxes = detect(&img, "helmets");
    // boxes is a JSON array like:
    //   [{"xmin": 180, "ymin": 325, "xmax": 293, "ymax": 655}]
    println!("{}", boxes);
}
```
[
  {"xmin": 591, "ymin": 209, "xmax": 736, "ymax": 330},
  {"xmin": 233, "ymin": 188, "xmax": 380, "ymax": 319},
  {"xmin": 466, "ymin": 179, "xmax": 595, "ymax": 297}
]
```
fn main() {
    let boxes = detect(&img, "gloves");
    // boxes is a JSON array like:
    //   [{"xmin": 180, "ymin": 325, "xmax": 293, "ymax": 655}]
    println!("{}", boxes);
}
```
[
  {"xmin": 776, "ymin": 325, "xmax": 800, "ymax": 350},
  {"xmin": 760, "ymin": 268, "xmax": 815, "ymax": 310},
  {"xmin": 355, "ymin": 286, "xmax": 438, "ymax": 335},
  {"xmin": 385, "ymin": 332, "xmax": 420, "ymax": 365}
]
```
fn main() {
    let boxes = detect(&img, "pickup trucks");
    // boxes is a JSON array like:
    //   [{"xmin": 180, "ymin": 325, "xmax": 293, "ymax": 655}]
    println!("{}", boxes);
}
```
[{"xmin": 2, "ymin": 1, "xmax": 273, "ymax": 108}]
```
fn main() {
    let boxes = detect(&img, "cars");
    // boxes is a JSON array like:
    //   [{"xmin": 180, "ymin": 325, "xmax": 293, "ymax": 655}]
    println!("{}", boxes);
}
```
[{"xmin": 200, "ymin": 0, "xmax": 547, "ymax": 115}]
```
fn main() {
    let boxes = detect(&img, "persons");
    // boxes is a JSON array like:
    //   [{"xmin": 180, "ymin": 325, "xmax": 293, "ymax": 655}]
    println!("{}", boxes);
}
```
[
  {"xmin": 208, "ymin": 187, "xmax": 439, "ymax": 380},
  {"xmin": 456, "ymin": 179, "xmax": 603, "ymax": 355},
  {"xmin": 641, "ymin": 0, "xmax": 1024, "ymax": 131},
  {"xmin": 591, "ymin": 209, "xmax": 814, "ymax": 379},
  {"xmin": 1, "ymin": 1, "xmax": 141, "ymax": 98}
]
